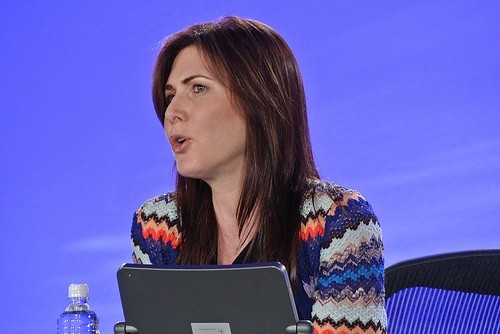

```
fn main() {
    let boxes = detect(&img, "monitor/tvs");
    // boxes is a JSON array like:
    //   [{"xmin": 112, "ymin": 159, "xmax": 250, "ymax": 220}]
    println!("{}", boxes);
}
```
[{"xmin": 114, "ymin": 263, "xmax": 312, "ymax": 334}]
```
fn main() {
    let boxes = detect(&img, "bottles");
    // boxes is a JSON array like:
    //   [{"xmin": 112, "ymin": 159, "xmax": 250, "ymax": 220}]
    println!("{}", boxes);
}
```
[{"xmin": 54, "ymin": 282, "xmax": 100, "ymax": 334}]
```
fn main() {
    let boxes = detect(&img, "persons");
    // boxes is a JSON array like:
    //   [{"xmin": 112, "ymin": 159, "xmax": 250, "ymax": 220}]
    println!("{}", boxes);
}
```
[{"xmin": 127, "ymin": 15, "xmax": 391, "ymax": 333}]
